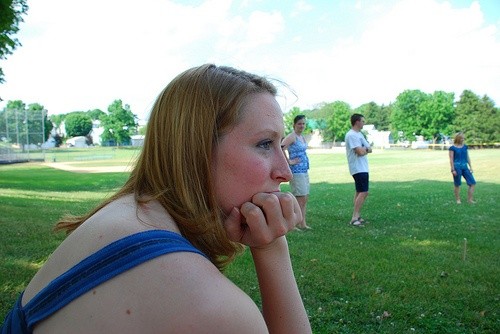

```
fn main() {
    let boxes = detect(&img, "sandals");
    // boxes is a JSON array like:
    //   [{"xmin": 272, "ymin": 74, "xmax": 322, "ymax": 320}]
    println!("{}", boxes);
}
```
[
  {"xmin": 350, "ymin": 220, "xmax": 365, "ymax": 228},
  {"xmin": 358, "ymin": 217, "xmax": 369, "ymax": 224}
]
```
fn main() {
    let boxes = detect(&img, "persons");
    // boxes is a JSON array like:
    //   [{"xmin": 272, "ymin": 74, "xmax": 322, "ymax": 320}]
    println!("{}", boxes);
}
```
[
  {"xmin": 345, "ymin": 113, "xmax": 372, "ymax": 226},
  {"xmin": 281, "ymin": 115, "xmax": 312, "ymax": 232},
  {"xmin": 448, "ymin": 134, "xmax": 477, "ymax": 204},
  {"xmin": 0, "ymin": 63, "xmax": 313, "ymax": 334}
]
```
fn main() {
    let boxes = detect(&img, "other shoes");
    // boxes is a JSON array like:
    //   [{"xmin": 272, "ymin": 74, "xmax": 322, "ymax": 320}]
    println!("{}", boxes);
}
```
[
  {"xmin": 456, "ymin": 200, "xmax": 461, "ymax": 204},
  {"xmin": 302, "ymin": 226, "xmax": 313, "ymax": 232},
  {"xmin": 292, "ymin": 226, "xmax": 303, "ymax": 233},
  {"xmin": 468, "ymin": 201, "xmax": 476, "ymax": 204}
]
